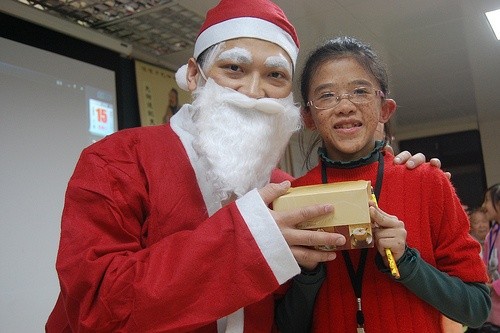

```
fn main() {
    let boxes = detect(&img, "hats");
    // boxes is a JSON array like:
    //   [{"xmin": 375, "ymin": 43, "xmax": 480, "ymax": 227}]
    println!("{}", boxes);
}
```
[{"xmin": 175, "ymin": 0, "xmax": 300, "ymax": 92}]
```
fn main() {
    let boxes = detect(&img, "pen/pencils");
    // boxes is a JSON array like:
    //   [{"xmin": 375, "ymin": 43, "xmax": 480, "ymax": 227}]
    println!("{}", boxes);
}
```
[{"xmin": 370, "ymin": 185, "xmax": 400, "ymax": 279}]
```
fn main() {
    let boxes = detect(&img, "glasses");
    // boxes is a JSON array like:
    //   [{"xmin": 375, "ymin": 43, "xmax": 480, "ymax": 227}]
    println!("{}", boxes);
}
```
[{"xmin": 306, "ymin": 87, "xmax": 386, "ymax": 110}]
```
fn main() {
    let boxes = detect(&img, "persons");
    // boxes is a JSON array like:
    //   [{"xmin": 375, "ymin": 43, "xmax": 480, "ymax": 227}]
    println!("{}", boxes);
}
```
[
  {"xmin": 46, "ymin": 0, "xmax": 451, "ymax": 333},
  {"xmin": 275, "ymin": 38, "xmax": 492, "ymax": 333},
  {"xmin": 443, "ymin": 184, "xmax": 500, "ymax": 333}
]
